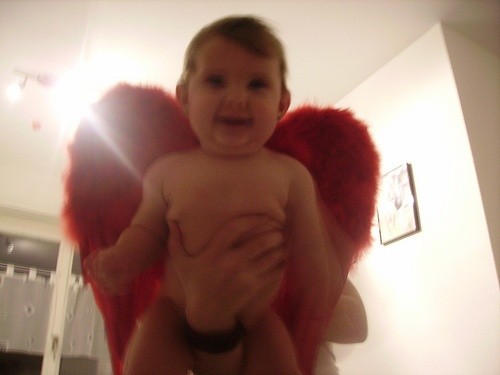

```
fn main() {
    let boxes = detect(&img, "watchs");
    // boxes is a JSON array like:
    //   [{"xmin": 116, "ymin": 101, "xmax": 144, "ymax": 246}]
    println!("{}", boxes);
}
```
[{"xmin": 178, "ymin": 313, "xmax": 244, "ymax": 357}]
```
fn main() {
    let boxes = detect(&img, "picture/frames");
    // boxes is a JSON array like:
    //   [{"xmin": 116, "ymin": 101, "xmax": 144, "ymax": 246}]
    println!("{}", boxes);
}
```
[{"xmin": 375, "ymin": 162, "xmax": 424, "ymax": 246}]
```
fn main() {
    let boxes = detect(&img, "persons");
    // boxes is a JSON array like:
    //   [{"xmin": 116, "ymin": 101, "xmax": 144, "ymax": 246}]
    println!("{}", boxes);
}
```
[
  {"xmin": 164, "ymin": 220, "xmax": 368, "ymax": 375},
  {"xmin": 82, "ymin": 14, "xmax": 342, "ymax": 375}
]
